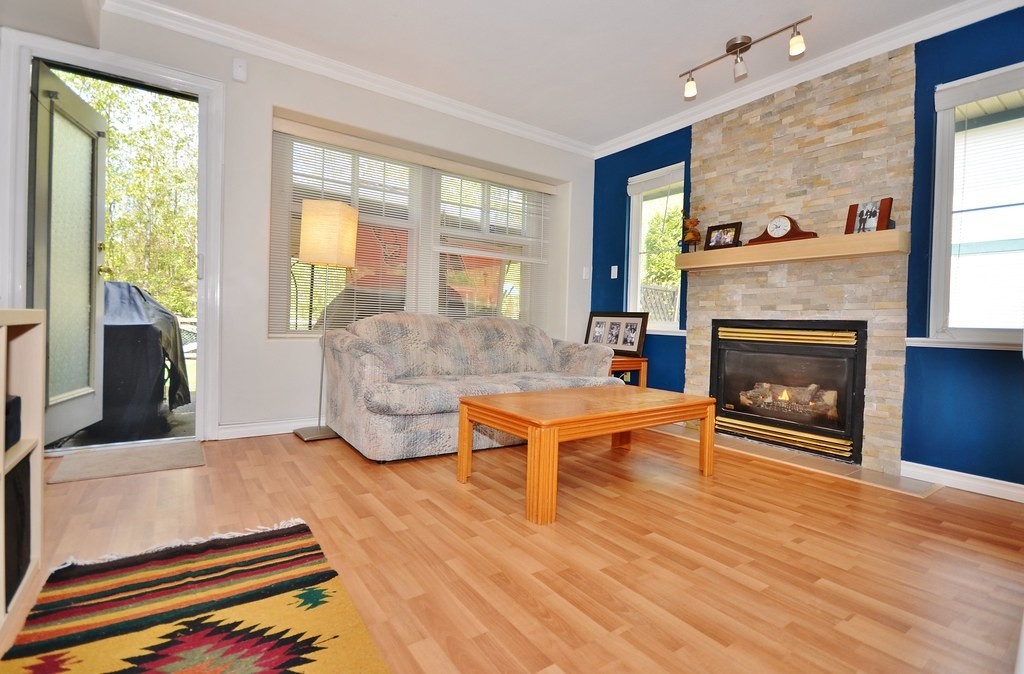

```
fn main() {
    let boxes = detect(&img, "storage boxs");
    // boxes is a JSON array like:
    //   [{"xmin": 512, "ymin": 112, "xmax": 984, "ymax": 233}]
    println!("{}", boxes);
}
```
[
  {"xmin": 6, "ymin": 394, "xmax": 22, "ymax": 452},
  {"xmin": 5, "ymin": 455, "xmax": 31, "ymax": 614}
]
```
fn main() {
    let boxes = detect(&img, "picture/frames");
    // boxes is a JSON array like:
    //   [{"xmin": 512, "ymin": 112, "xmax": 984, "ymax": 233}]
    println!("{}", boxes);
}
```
[
  {"xmin": 583, "ymin": 311, "xmax": 649, "ymax": 357},
  {"xmin": 703, "ymin": 222, "xmax": 743, "ymax": 250}
]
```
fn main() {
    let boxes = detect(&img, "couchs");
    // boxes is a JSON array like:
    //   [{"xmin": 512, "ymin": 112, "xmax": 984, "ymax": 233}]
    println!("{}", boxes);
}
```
[{"xmin": 319, "ymin": 312, "xmax": 626, "ymax": 466}]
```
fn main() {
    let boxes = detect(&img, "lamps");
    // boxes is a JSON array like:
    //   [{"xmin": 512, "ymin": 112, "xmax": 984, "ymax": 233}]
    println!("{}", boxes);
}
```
[
  {"xmin": 294, "ymin": 198, "xmax": 359, "ymax": 444},
  {"xmin": 675, "ymin": 15, "xmax": 813, "ymax": 98}
]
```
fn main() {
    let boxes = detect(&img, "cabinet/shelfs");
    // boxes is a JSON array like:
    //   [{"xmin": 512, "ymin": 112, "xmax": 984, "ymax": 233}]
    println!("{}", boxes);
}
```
[{"xmin": 0, "ymin": 309, "xmax": 42, "ymax": 629}]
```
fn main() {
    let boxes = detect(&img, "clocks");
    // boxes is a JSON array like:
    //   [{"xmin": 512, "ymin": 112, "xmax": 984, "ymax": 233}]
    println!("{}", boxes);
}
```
[{"xmin": 743, "ymin": 215, "xmax": 818, "ymax": 246}]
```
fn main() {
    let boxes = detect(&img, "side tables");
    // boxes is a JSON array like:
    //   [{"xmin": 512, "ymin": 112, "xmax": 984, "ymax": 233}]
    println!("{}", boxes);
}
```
[{"xmin": 609, "ymin": 356, "xmax": 649, "ymax": 388}]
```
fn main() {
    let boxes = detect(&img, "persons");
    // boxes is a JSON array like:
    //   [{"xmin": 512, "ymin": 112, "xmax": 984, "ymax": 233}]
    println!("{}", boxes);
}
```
[
  {"xmin": 593, "ymin": 321, "xmax": 637, "ymax": 347},
  {"xmin": 858, "ymin": 205, "xmax": 877, "ymax": 233},
  {"xmin": 714, "ymin": 228, "xmax": 731, "ymax": 245}
]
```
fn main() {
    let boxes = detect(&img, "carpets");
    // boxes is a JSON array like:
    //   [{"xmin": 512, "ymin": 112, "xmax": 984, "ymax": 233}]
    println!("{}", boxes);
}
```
[
  {"xmin": 0, "ymin": 517, "xmax": 396, "ymax": 674},
  {"xmin": 48, "ymin": 441, "xmax": 207, "ymax": 485}
]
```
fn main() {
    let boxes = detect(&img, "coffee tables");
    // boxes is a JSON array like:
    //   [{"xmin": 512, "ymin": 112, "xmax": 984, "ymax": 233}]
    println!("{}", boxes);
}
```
[{"xmin": 455, "ymin": 385, "xmax": 716, "ymax": 526}]
